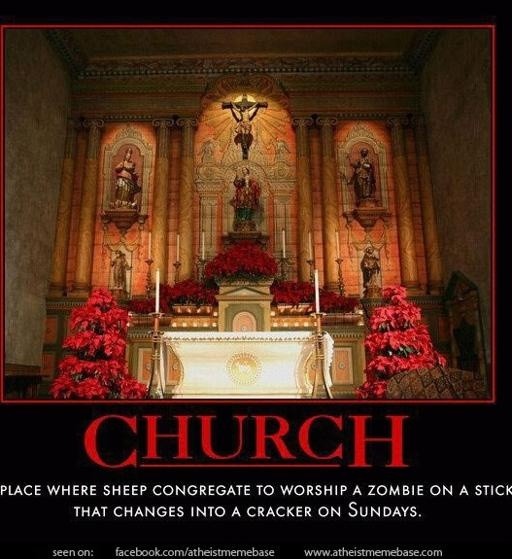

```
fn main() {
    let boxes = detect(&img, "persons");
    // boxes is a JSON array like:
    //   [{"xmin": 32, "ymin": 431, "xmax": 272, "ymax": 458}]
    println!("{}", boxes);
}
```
[
  {"xmin": 230, "ymin": 100, "xmax": 259, "ymax": 154},
  {"xmin": 110, "ymin": 250, "xmax": 127, "ymax": 288},
  {"xmin": 233, "ymin": 167, "xmax": 254, "ymax": 221},
  {"xmin": 112, "ymin": 148, "xmax": 142, "ymax": 201},
  {"xmin": 360, "ymin": 246, "xmax": 380, "ymax": 299},
  {"xmin": 347, "ymin": 148, "xmax": 373, "ymax": 200}
]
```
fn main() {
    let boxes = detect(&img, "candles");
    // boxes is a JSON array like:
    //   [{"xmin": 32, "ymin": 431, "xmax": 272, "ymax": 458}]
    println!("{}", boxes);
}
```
[
  {"xmin": 148, "ymin": 232, "xmax": 152, "ymax": 260},
  {"xmin": 335, "ymin": 231, "xmax": 340, "ymax": 259},
  {"xmin": 314, "ymin": 269, "xmax": 320, "ymax": 314},
  {"xmin": 282, "ymin": 230, "xmax": 286, "ymax": 259},
  {"xmin": 308, "ymin": 232, "xmax": 312, "ymax": 260},
  {"xmin": 202, "ymin": 231, "xmax": 205, "ymax": 259},
  {"xmin": 156, "ymin": 268, "xmax": 160, "ymax": 314},
  {"xmin": 177, "ymin": 235, "xmax": 179, "ymax": 262}
]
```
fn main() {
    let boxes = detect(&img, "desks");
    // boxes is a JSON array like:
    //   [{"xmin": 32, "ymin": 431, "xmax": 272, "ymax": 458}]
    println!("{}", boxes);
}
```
[{"xmin": 156, "ymin": 331, "xmax": 334, "ymax": 399}]
